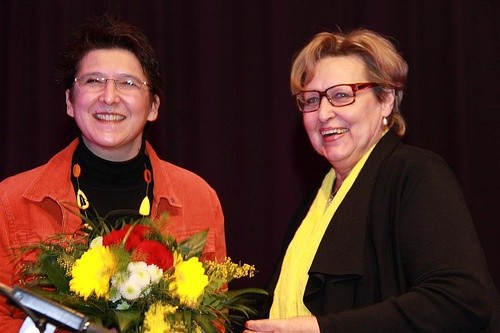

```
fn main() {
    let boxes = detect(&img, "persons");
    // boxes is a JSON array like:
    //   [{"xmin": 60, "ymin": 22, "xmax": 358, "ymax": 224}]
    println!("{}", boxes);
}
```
[
  {"xmin": 0, "ymin": 18, "xmax": 228, "ymax": 333},
  {"xmin": 242, "ymin": 30, "xmax": 500, "ymax": 333}
]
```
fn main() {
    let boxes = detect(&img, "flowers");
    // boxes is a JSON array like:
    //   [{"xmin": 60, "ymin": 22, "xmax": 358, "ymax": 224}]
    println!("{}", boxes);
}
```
[{"xmin": 4, "ymin": 200, "xmax": 269, "ymax": 333}]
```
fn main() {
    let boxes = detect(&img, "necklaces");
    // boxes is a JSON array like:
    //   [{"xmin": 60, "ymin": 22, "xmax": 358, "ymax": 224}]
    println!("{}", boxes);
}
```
[
  {"xmin": 325, "ymin": 172, "xmax": 350, "ymax": 211},
  {"xmin": 72, "ymin": 142, "xmax": 151, "ymax": 241}
]
